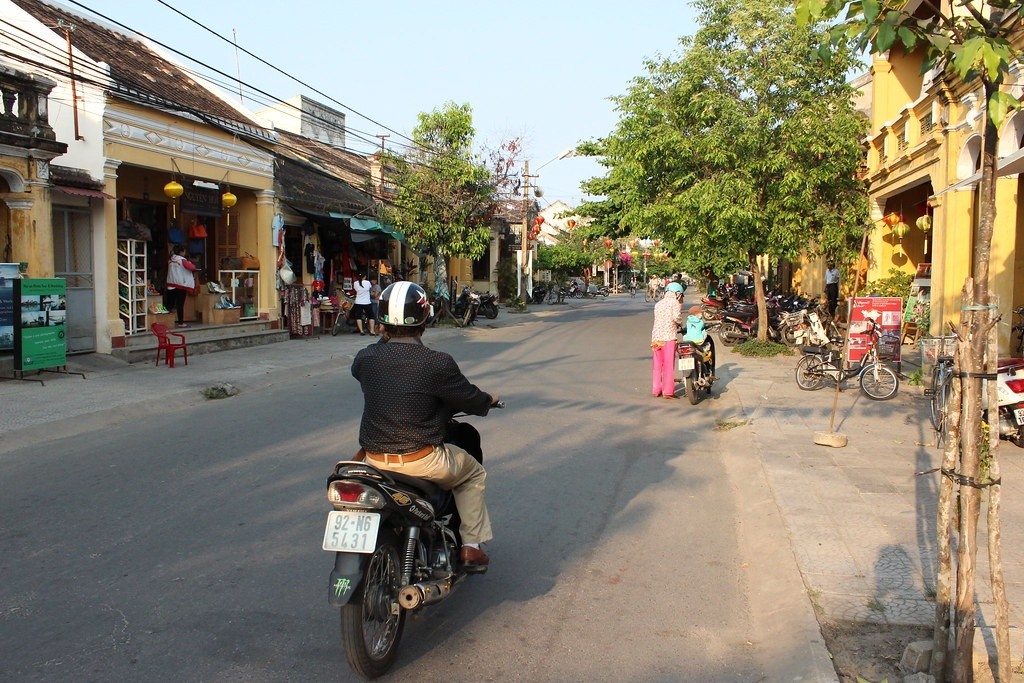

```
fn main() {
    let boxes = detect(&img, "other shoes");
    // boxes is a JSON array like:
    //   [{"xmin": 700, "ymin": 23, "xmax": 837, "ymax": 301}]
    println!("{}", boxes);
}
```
[
  {"xmin": 663, "ymin": 393, "xmax": 679, "ymax": 399},
  {"xmin": 370, "ymin": 333, "xmax": 380, "ymax": 336},
  {"xmin": 361, "ymin": 332, "xmax": 366, "ymax": 335},
  {"xmin": 178, "ymin": 324, "xmax": 191, "ymax": 327}
]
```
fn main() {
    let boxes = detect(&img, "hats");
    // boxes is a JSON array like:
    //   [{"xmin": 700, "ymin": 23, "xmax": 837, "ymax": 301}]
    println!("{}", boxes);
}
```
[{"xmin": 688, "ymin": 306, "xmax": 704, "ymax": 314}]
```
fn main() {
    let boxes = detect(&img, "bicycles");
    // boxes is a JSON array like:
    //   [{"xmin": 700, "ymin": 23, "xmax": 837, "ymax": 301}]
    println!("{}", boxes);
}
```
[
  {"xmin": 920, "ymin": 330, "xmax": 959, "ymax": 445},
  {"xmin": 332, "ymin": 287, "xmax": 355, "ymax": 336},
  {"xmin": 630, "ymin": 286, "xmax": 638, "ymax": 299},
  {"xmin": 793, "ymin": 316, "xmax": 904, "ymax": 400},
  {"xmin": 645, "ymin": 285, "xmax": 666, "ymax": 304}
]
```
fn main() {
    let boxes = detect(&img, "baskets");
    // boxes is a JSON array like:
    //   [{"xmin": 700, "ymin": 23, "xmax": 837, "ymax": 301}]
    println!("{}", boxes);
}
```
[
  {"xmin": 919, "ymin": 338, "xmax": 957, "ymax": 364},
  {"xmin": 878, "ymin": 335, "xmax": 900, "ymax": 356},
  {"xmin": 786, "ymin": 312, "xmax": 804, "ymax": 327}
]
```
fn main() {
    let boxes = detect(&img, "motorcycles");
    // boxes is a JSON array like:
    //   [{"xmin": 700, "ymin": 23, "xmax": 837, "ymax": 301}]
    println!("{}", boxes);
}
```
[
  {"xmin": 319, "ymin": 400, "xmax": 509, "ymax": 678},
  {"xmin": 675, "ymin": 321, "xmax": 721, "ymax": 405}
]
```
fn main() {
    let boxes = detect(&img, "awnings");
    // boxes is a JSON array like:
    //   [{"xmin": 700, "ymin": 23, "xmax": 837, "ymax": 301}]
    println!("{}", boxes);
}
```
[{"xmin": 55, "ymin": 185, "xmax": 116, "ymax": 200}]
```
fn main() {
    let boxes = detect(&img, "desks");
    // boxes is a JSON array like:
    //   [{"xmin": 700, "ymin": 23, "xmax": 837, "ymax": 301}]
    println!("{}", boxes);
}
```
[{"xmin": 321, "ymin": 310, "xmax": 338, "ymax": 334}]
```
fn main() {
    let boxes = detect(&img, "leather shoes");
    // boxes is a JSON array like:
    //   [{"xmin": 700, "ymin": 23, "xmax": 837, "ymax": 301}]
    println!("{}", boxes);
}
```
[{"xmin": 461, "ymin": 545, "xmax": 490, "ymax": 566}]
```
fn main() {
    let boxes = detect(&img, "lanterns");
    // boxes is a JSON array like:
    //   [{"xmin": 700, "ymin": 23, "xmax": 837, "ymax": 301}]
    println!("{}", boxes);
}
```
[
  {"xmin": 567, "ymin": 219, "xmax": 575, "ymax": 238},
  {"xmin": 883, "ymin": 212, "xmax": 905, "ymax": 246},
  {"xmin": 164, "ymin": 180, "xmax": 183, "ymax": 218},
  {"xmin": 528, "ymin": 216, "xmax": 544, "ymax": 243},
  {"xmin": 892, "ymin": 222, "xmax": 910, "ymax": 257},
  {"xmin": 606, "ymin": 241, "xmax": 612, "ymax": 253},
  {"xmin": 222, "ymin": 192, "xmax": 237, "ymax": 226},
  {"xmin": 916, "ymin": 215, "xmax": 933, "ymax": 255},
  {"xmin": 654, "ymin": 240, "xmax": 659, "ymax": 250}
]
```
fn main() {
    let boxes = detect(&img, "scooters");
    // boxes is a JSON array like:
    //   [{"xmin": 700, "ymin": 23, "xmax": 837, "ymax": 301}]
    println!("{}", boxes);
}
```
[
  {"xmin": 525, "ymin": 279, "xmax": 610, "ymax": 304},
  {"xmin": 450, "ymin": 275, "xmax": 499, "ymax": 327},
  {"xmin": 982, "ymin": 356, "xmax": 1024, "ymax": 447},
  {"xmin": 699, "ymin": 271, "xmax": 843, "ymax": 354}
]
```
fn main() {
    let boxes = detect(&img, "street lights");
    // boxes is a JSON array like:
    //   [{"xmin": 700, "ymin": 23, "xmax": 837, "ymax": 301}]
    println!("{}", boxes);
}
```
[{"xmin": 518, "ymin": 147, "xmax": 575, "ymax": 312}]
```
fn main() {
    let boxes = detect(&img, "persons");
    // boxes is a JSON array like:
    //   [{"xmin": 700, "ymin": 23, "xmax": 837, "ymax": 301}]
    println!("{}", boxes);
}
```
[
  {"xmin": 351, "ymin": 281, "xmax": 499, "ymax": 568},
  {"xmin": 647, "ymin": 273, "xmax": 685, "ymax": 299},
  {"xmin": 629, "ymin": 275, "xmax": 636, "ymax": 293},
  {"xmin": 652, "ymin": 282, "xmax": 683, "ymax": 399},
  {"xmin": 354, "ymin": 272, "xmax": 379, "ymax": 336},
  {"xmin": 683, "ymin": 306, "xmax": 706, "ymax": 345},
  {"xmin": 163, "ymin": 244, "xmax": 196, "ymax": 328},
  {"xmin": 824, "ymin": 260, "xmax": 840, "ymax": 319},
  {"xmin": 369, "ymin": 276, "xmax": 381, "ymax": 333}
]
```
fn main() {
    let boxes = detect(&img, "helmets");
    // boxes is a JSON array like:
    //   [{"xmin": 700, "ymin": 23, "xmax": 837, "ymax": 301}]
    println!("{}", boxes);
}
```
[
  {"xmin": 665, "ymin": 282, "xmax": 684, "ymax": 298},
  {"xmin": 376, "ymin": 280, "xmax": 432, "ymax": 326}
]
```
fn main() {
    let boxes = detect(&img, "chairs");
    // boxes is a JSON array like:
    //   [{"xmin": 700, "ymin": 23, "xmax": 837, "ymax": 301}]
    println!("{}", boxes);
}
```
[{"xmin": 150, "ymin": 322, "xmax": 188, "ymax": 368}]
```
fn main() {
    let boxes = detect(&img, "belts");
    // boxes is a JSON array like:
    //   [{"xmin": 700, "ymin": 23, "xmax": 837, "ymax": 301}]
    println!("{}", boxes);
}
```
[{"xmin": 365, "ymin": 444, "xmax": 435, "ymax": 463}]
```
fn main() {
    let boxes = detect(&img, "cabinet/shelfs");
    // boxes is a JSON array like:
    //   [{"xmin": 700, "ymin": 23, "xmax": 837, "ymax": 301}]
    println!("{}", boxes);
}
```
[
  {"xmin": 217, "ymin": 270, "xmax": 261, "ymax": 321},
  {"xmin": 117, "ymin": 239, "xmax": 175, "ymax": 335},
  {"xmin": 202, "ymin": 293, "xmax": 240, "ymax": 324}
]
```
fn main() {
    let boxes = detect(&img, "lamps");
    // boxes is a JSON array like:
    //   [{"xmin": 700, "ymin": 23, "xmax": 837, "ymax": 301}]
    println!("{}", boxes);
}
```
[{"xmin": 192, "ymin": 180, "xmax": 219, "ymax": 189}]
